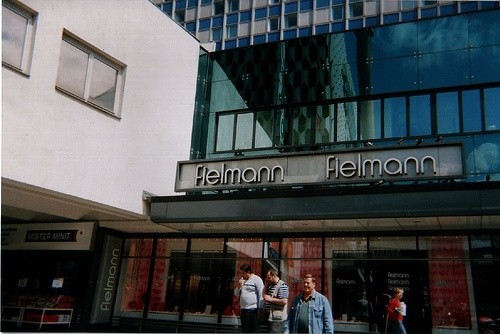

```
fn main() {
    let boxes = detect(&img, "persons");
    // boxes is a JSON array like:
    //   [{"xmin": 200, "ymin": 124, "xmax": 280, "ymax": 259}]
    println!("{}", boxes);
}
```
[
  {"xmin": 262, "ymin": 268, "xmax": 289, "ymax": 333},
  {"xmin": 388, "ymin": 287, "xmax": 409, "ymax": 334},
  {"xmin": 288, "ymin": 274, "xmax": 335, "ymax": 334},
  {"xmin": 234, "ymin": 264, "xmax": 265, "ymax": 333}
]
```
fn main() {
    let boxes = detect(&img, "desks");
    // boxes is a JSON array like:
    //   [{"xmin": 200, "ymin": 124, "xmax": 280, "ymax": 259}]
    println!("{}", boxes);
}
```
[{"xmin": 1, "ymin": 304, "xmax": 74, "ymax": 332}]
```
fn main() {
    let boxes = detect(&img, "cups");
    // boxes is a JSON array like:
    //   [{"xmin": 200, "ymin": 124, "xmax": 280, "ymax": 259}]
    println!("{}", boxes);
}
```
[{"xmin": 205, "ymin": 305, "xmax": 213, "ymax": 315}]
